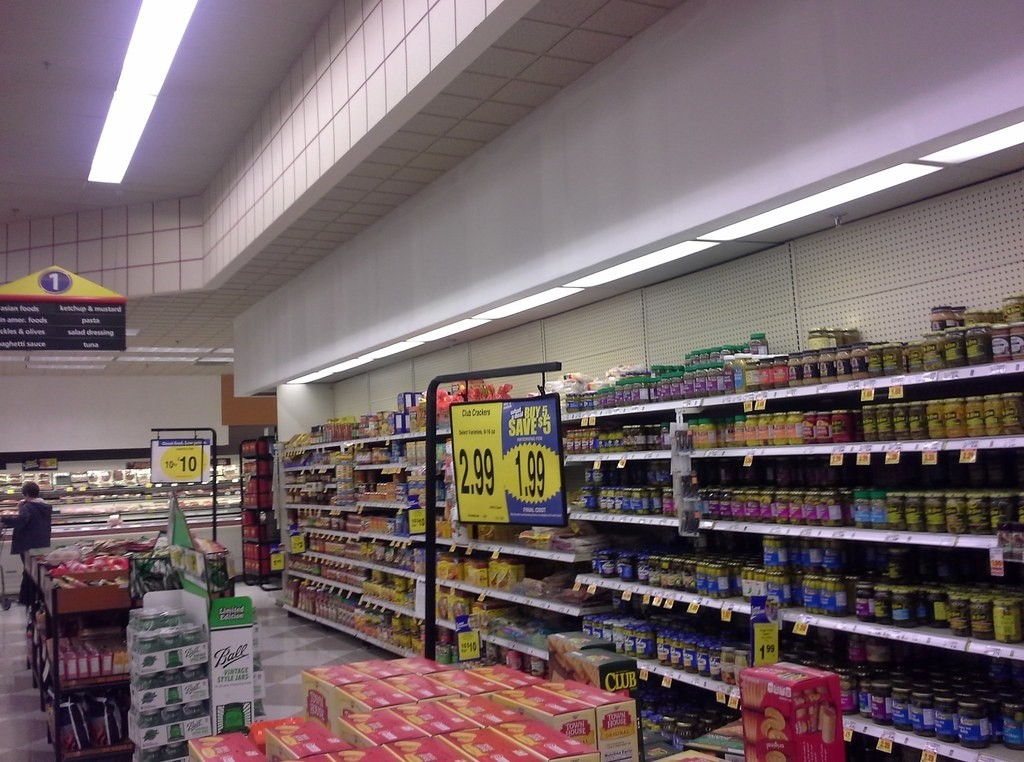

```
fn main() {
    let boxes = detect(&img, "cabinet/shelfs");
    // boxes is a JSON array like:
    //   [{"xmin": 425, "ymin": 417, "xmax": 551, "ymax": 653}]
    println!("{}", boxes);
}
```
[
  {"xmin": 283, "ymin": 428, "xmax": 613, "ymax": 661},
  {"xmin": 239, "ymin": 435, "xmax": 284, "ymax": 591},
  {"xmin": 27, "ymin": 552, "xmax": 143, "ymax": 762},
  {"xmin": 562, "ymin": 359, "xmax": 1024, "ymax": 762}
]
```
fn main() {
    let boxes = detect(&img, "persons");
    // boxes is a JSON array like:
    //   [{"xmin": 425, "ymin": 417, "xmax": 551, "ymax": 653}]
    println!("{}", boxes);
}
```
[{"xmin": 1, "ymin": 482, "xmax": 53, "ymax": 605}]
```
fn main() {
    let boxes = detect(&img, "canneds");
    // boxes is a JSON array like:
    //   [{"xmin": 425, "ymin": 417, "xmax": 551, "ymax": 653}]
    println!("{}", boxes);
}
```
[
  {"xmin": 566, "ymin": 296, "xmax": 1024, "ymax": 753},
  {"xmin": 127, "ymin": 608, "xmax": 207, "ymax": 762}
]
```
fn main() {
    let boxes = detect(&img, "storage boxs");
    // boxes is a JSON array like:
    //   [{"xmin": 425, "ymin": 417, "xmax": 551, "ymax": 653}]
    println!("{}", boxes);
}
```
[
  {"xmin": 562, "ymin": 649, "xmax": 645, "ymax": 762},
  {"xmin": 381, "ymin": 736, "xmax": 474, "ymax": 762},
  {"xmin": 25, "ymin": 551, "xmax": 402, "ymax": 762},
  {"xmin": 437, "ymin": 694, "xmax": 528, "ymax": 730},
  {"xmin": 491, "ymin": 685, "xmax": 597, "ymax": 749},
  {"xmin": 385, "ymin": 656, "xmax": 458, "ymax": 676},
  {"xmin": 285, "ymin": 390, "xmax": 555, "ymax": 550},
  {"xmin": 464, "ymin": 664, "xmax": 549, "ymax": 690},
  {"xmin": 532, "ymin": 679, "xmax": 639, "ymax": 762},
  {"xmin": 343, "ymin": 659, "xmax": 416, "ymax": 681},
  {"xmin": 378, "ymin": 673, "xmax": 460, "ymax": 703},
  {"xmin": 337, "ymin": 709, "xmax": 429, "ymax": 748},
  {"xmin": 437, "ymin": 728, "xmax": 544, "ymax": 762},
  {"xmin": 387, "ymin": 701, "xmax": 482, "ymax": 738},
  {"xmin": 332, "ymin": 680, "xmax": 417, "ymax": 738},
  {"xmin": 423, "ymin": 670, "xmax": 510, "ymax": 700},
  {"xmin": 436, "ymin": 553, "xmax": 616, "ymax": 683},
  {"xmin": 487, "ymin": 718, "xmax": 601, "ymax": 762},
  {"xmin": 656, "ymin": 719, "xmax": 745, "ymax": 762},
  {"xmin": 738, "ymin": 662, "xmax": 846, "ymax": 762}
]
